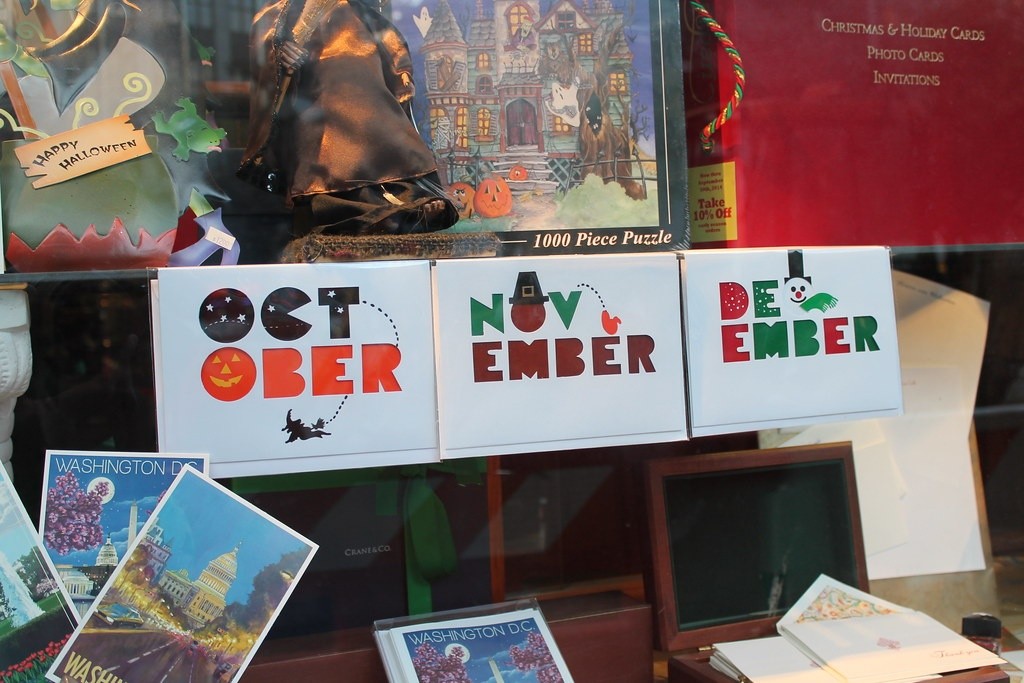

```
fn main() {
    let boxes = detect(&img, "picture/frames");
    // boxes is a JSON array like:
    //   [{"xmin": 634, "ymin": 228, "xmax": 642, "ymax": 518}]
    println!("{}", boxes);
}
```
[{"xmin": 648, "ymin": 443, "xmax": 871, "ymax": 651}]
